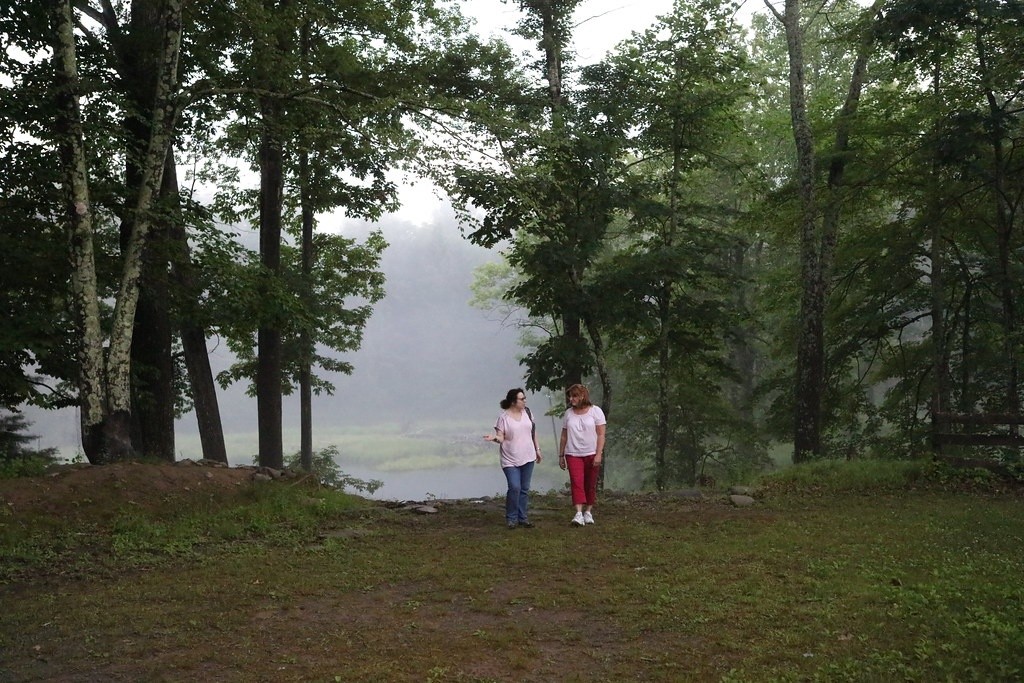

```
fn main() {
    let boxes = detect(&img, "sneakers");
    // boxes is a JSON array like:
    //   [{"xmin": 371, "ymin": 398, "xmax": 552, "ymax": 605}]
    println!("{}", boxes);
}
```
[
  {"xmin": 520, "ymin": 521, "xmax": 535, "ymax": 528},
  {"xmin": 584, "ymin": 512, "xmax": 594, "ymax": 524},
  {"xmin": 507, "ymin": 521, "xmax": 519, "ymax": 529},
  {"xmin": 571, "ymin": 514, "xmax": 584, "ymax": 527}
]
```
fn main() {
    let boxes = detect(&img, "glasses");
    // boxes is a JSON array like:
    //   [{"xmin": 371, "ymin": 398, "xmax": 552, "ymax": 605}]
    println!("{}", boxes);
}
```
[{"xmin": 518, "ymin": 396, "xmax": 527, "ymax": 400}]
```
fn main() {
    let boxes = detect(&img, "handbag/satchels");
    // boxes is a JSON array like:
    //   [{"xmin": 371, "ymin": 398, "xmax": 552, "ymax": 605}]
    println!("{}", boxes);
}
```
[{"xmin": 524, "ymin": 406, "xmax": 536, "ymax": 439}]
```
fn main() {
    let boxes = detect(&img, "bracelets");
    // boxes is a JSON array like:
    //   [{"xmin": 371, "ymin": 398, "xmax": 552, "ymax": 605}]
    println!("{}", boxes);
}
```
[
  {"xmin": 536, "ymin": 448, "xmax": 541, "ymax": 450},
  {"xmin": 559, "ymin": 455, "xmax": 564, "ymax": 457}
]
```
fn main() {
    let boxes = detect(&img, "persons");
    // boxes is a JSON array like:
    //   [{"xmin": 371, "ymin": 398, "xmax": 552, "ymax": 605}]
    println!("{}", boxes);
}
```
[
  {"xmin": 483, "ymin": 388, "xmax": 542, "ymax": 529},
  {"xmin": 559, "ymin": 384, "xmax": 606, "ymax": 526}
]
